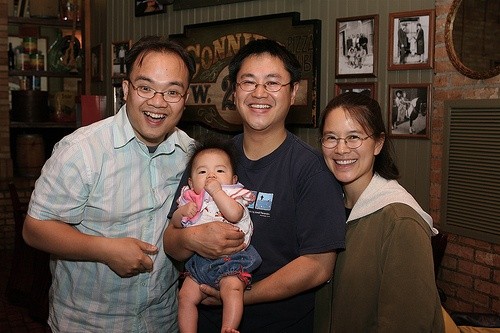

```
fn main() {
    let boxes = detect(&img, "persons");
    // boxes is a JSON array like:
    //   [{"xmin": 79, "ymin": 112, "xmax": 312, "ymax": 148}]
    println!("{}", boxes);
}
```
[
  {"xmin": 398, "ymin": 22, "xmax": 424, "ymax": 64},
  {"xmin": 162, "ymin": 38, "xmax": 347, "ymax": 333},
  {"xmin": 23, "ymin": 35, "xmax": 201, "ymax": 333},
  {"xmin": 302, "ymin": 91, "xmax": 446, "ymax": 333},
  {"xmin": 347, "ymin": 33, "xmax": 368, "ymax": 69},
  {"xmin": 392, "ymin": 90, "xmax": 426, "ymax": 135},
  {"xmin": 172, "ymin": 144, "xmax": 263, "ymax": 333}
]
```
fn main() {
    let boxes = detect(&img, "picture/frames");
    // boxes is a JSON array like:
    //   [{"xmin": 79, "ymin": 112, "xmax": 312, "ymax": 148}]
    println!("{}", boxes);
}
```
[
  {"xmin": 335, "ymin": 14, "xmax": 378, "ymax": 78},
  {"xmin": 334, "ymin": 81, "xmax": 377, "ymax": 102},
  {"xmin": 135, "ymin": 0, "xmax": 167, "ymax": 17},
  {"xmin": 387, "ymin": 9, "xmax": 435, "ymax": 70},
  {"xmin": 388, "ymin": 83, "xmax": 431, "ymax": 140},
  {"xmin": 91, "ymin": 44, "xmax": 104, "ymax": 82}
]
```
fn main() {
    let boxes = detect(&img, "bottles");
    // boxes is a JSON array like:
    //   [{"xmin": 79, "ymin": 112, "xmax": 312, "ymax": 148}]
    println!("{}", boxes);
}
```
[{"xmin": 7, "ymin": 36, "xmax": 44, "ymax": 70}]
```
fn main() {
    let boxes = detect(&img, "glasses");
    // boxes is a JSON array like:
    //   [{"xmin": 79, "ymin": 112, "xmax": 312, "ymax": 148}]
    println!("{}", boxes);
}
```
[
  {"xmin": 124, "ymin": 76, "xmax": 190, "ymax": 103},
  {"xmin": 320, "ymin": 132, "xmax": 377, "ymax": 149},
  {"xmin": 234, "ymin": 79, "xmax": 294, "ymax": 92}
]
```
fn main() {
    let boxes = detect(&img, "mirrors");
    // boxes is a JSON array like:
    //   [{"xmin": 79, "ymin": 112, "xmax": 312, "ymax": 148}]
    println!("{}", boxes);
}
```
[{"xmin": 444, "ymin": 0, "xmax": 500, "ymax": 80}]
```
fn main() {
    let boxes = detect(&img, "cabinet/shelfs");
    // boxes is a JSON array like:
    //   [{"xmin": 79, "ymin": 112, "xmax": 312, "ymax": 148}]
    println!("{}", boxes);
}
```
[{"xmin": 7, "ymin": 0, "xmax": 90, "ymax": 179}]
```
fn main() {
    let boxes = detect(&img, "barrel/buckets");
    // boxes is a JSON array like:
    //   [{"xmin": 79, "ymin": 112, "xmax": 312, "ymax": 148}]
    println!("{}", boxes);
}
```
[
  {"xmin": 50, "ymin": 90, "xmax": 76, "ymax": 121},
  {"xmin": 11, "ymin": 90, "xmax": 48, "ymax": 121},
  {"xmin": 16, "ymin": 134, "xmax": 44, "ymax": 178}
]
